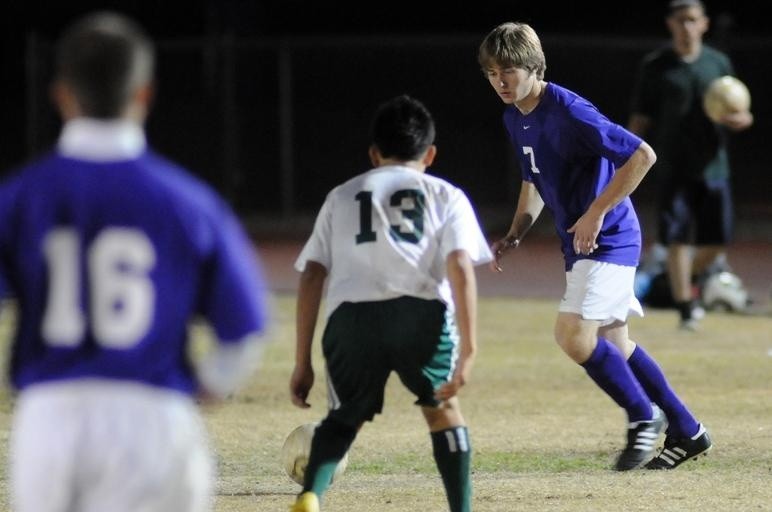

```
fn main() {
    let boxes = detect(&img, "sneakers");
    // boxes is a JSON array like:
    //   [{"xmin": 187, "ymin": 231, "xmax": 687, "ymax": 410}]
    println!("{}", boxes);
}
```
[
  {"xmin": 288, "ymin": 491, "xmax": 320, "ymax": 512},
  {"xmin": 611, "ymin": 396, "xmax": 671, "ymax": 472},
  {"xmin": 647, "ymin": 422, "xmax": 714, "ymax": 477}
]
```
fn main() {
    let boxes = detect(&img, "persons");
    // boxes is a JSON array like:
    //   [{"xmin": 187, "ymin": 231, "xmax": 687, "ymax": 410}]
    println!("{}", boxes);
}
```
[
  {"xmin": 0, "ymin": 11, "xmax": 276, "ymax": 512},
  {"xmin": 628, "ymin": 1, "xmax": 756, "ymax": 334},
  {"xmin": 477, "ymin": 20, "xmax": 712, "ymax": 472},
  {"xmin": 286, "ymin": 91, "xmax": 497, "ymax": 510}
]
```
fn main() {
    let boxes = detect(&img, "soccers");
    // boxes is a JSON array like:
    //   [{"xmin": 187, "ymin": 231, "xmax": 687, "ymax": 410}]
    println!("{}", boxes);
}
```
[
  {"xmin": 702, "ymin": 271, "xmax": 747, "ymax": 315},
  {"xmin": 281, "ymin": 423, "xmax": 349, "ymax": 489},
  {"xmin": 704, "ymin": 76, "xmax": 751, "ymax": 123}
]
